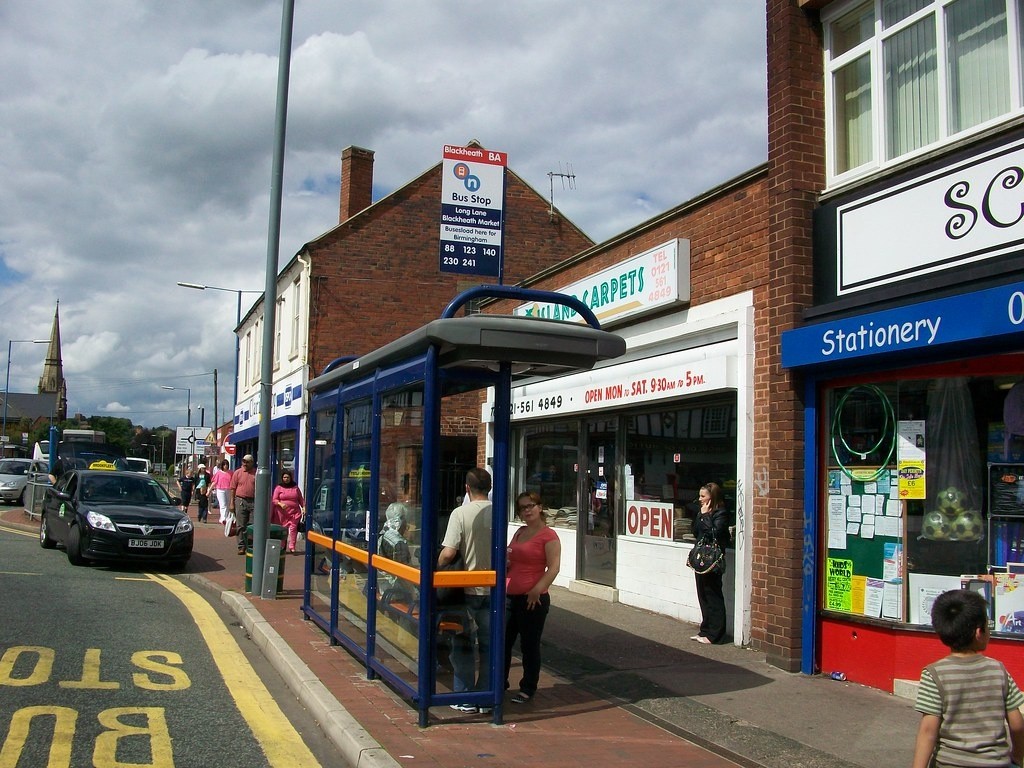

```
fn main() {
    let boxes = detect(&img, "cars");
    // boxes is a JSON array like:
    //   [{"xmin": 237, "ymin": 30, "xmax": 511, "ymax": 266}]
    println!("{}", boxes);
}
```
[
  {"xmin": 0, "ymin": 458, "xmax": 49, "ymax": 507},
  {"xmin": 39, "ymin": 460, "xmax": 194, "ymax": 574},
  {"xmin": 312, "ymin": 469, "xmax": 406, "ymax": 554},
  {"xmin": 526, "ymin": 473, "xmax": 571, "ymax": 510},
  {"xmin": 686, "ymin": 479, "xmax": 736, "ymax": 524}
]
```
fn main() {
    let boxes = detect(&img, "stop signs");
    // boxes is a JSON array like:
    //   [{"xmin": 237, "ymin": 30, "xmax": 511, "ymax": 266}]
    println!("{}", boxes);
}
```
[{"xmin": 223, "ymin": 433, "xmax": 237, "ymax": 456}]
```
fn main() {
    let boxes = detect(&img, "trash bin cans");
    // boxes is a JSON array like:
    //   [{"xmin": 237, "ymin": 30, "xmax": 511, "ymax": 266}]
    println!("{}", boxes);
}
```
[{"xmin": 243, "ymin": 524, "xmax": 289, "ymax": 592}]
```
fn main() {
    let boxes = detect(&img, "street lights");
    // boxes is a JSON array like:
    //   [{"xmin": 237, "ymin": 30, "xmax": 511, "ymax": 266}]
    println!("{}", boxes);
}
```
[
  {"xmin": 161, "ymin": 386, "xmax": 190, "ymax": 470},
  {"xmin": 130, "ymin": 449, "xmax": 134, "ymax": 454},
  {"xmin": 141, "ymin": 443, "xmax": 156, "ymax": 470},
  {"xmin": 176, "ymin": 280, "xmax": 265, "ymax": 472},
  {"xmin": 1, "ymin": 339, "xmax": 52, "ymax": 458},
  {"xmin": 151, "ymin": 434, "xmax": 164, "ymax": 472}
]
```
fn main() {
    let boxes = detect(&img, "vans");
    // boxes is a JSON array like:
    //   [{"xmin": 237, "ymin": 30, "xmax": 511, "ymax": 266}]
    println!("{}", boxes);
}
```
[{"xmin": 126, "ymin": 456, "xmax": 154, "ymax": 476}]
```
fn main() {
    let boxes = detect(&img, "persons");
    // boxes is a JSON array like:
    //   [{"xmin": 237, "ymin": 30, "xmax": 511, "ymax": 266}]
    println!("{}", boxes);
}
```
[
  {"xmin": 689, "ymin": 482, "xmax": 731, "ymax": 643},
  {"xmin": 912, "ymin": 589, "xmax": 1024, "ymax": 768},
  {"xmin": 272, "ymin": 471, "xmax": 306, "ymax": 557},
  {"xmin": 591, "ymin": 490, "xmax": 601, "ymax": 515},
  {"xmin": 376, "ymin": 502, "xmax": 419, "ymax": 601},
  {"xmin": 197, "ymin": 485, "xmax": 208, "ymax": 523},
  {"xmin": 195, "ymin": 463, "xmax": 212, "ymax": 514},
  {"xmin": 213, "ymin": 460, "xmax": 222, "ymax": 502},
  {"xmin": 205, "ymin": 459, "xmax": 238, "ymax": 526},
  {"xmin": 48, "ymin": 442, "xmax": 76, "ymax": 486},
  {"xmin": 84, "ymin": 481, "xmax": 102, "ymax": 499},
  {"xmin": 176, "ymin": 469, "xmax": 196, "ymax": 514},
  {"xmin": 504, "ymin": 490, "xmax": 561, "ymax": 705},
  {"xmin": 436, "ymin": 467, "xmax": 492, "ymax": 714},
  {"xmin": 229, "ymin": 455, "xmax": 256, "ymax": 555}
]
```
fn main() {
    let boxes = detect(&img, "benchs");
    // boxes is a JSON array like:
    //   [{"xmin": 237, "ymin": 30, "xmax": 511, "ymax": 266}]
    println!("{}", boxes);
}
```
[{"xmin": 318, "ymin": 549, "xmax": 463, "ymax": 676}]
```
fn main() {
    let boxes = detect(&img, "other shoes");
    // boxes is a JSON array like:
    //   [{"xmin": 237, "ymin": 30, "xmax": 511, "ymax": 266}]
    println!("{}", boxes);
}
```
[
  {"xmin": 198, "ymin": 518, "xmax": 201, "ymax": 522},
  {"xmin": 511, "ymin": 693, "xmax": 531, "ymax": 703},
  {"xmin": 689, "ymin": 635, "xmax": 699, "ymax": 640},
  {"xmin": 450, "ymin": 705, "xmax": 477, "ymax": 713},
  {"xmin": 479, "ymin": 705, "xmax": 492, "ymax": 714},
  {"xmin": 203, "ymin": 520, "xmax": 207, "ymax": 522},
  {"xmin": 291, "ymin": 549, "xmax": 297, "ymax": 555},
  {"xmin": 697, "ymin": 636, "xmax": 710, "ymax": 643},
  {"xmin": 238, "ymin": 549, "xmax": 244, "ymax": 554}
]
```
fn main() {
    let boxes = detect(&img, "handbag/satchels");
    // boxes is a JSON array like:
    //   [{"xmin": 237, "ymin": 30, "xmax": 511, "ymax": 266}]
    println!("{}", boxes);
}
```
[
  {"xmin": 225, "ymin": 512, "xmax": 236, "ymax": 538},
  {"xmin": 298, "ymin": 514, "xmax": 306, "ymax": 532},
  {"xmin": 686, "ymin": 527, "xmax": 726, "ymax": 574}
]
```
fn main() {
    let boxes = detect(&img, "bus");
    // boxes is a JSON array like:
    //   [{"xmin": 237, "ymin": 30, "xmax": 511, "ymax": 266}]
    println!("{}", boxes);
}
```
[
  {"xmin": 0, "ymin": 444, "xmax": 29, "ymax": 458},
  {"xmin": 62, "ymin": 428, "xmax": 107, "ymax": 444}
]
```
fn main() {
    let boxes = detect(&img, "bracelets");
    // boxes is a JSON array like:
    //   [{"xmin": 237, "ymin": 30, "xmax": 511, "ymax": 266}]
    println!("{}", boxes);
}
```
[{"xmin": 192, "ymin": 489, "xmax": 195, "ymax": 491}]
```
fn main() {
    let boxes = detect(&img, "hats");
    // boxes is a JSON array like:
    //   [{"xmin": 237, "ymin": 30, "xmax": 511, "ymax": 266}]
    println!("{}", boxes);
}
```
[
  {"xmin": 243, "ymin": 454, "xmax": 254, "ymax": 464},
  {"xmin": 197, "ymin": 464, "xmax": 206, "ymax": 469}
]
